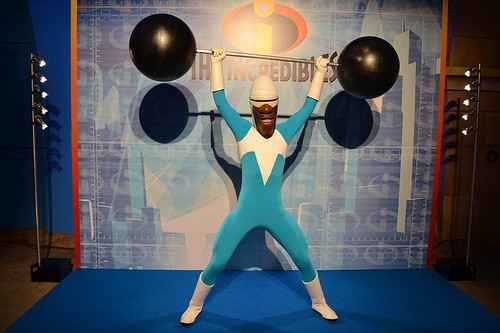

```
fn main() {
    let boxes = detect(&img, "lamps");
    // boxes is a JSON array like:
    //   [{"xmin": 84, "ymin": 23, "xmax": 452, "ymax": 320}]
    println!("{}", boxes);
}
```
[
  {"xmin": 461, "ymin": 66, "xmax": 478, "ymax": 136},
  {"xmin": 29, "ymin": 53, "xmax": 49, "ymax": 131}
]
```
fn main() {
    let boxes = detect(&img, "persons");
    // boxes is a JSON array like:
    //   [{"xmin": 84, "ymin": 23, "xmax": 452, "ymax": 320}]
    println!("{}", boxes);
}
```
[{"xmin": 180, "ymin": 46, "xmax": 339, "ymax": 324}]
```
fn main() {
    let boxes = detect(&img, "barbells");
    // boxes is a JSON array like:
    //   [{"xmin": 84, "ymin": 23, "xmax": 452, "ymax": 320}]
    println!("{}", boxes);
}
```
[{"xmin": 128, "ymin": 13, "xmax": 400, "ymax": 100}]
